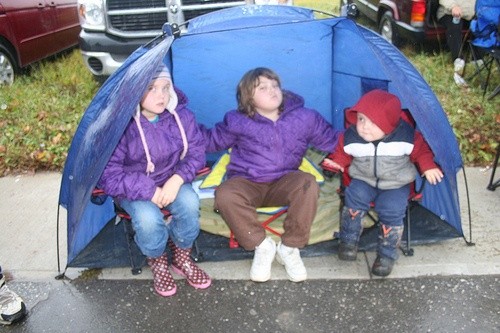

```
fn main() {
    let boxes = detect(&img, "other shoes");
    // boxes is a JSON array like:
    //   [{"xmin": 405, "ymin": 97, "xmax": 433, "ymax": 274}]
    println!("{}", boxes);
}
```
[
  {"xmin": 249, "ymin": 237, "xmax": 276, "ymax": 282},
  {"xmin": 276, "ymin": 240, "xmax": 308, "ymax": 282},
  {"xmin": 454, "ymin": 59, "xmax": 465, "ymax": 72},
  {"xmin": 0, "ymin": 274, "xmax": 29, "ymax": 326},
  {"xmin": 372, "ymin": 255, "xmax": 394, "ymax": 276},
  {"xmin": 339, "ymin": 242, "xmax": 358, "ymax": 261}
]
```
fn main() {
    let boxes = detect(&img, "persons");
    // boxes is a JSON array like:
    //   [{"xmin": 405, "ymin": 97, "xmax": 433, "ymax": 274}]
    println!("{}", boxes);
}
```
[
  {"xmin": 98, "ymin": 63, "xmax": 211, "ymax": 295},
  {"xmin": 318, "ymin": 89, "xmax": 443, "ymax": 276},
  {"xmin": 0, "ymin": 266, "xmax": 28, "ymax": 325},
  {"xmin": 199, "ymin": 67, "xmax": 339, "ymax": 282},
  {"xmin": 438, "ymin": 0, "xmax": 484, "ymax": 71}
]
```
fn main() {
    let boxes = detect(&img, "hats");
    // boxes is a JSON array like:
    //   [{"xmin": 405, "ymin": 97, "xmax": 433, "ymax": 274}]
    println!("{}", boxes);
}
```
[{"xmin": 131, "ymin": 62, "xmax": 189, "ymax": 174}]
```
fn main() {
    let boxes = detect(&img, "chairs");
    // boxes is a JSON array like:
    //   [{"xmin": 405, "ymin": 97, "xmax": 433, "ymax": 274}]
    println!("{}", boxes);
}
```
[
  {"xmin": 198, "ymin": 147, "xmax": 326, "ymax": 252},
  {"xmin": 426, "ymin": 0, "xmax": 474, "ymax": 79},
  {"xmin": 461, "ymin": 0, "xmax": 500, "ymax": 98},
  {"xmin": 320, "ymin": 106, "xmax": 428, "ymax": 258},
  {"xmin": 88, "ymin": 162, "xmax": 212, "ymax": 275}
]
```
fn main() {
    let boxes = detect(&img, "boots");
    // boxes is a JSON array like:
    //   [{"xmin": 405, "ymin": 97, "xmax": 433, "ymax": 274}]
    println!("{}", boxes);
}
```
[
  {"xmin": 169, "ymin": 239, "xmax": 211, "ymax": 288},
  {"xmin": 145, "ymin": 252, "xmax": 176, "ymax": 295}
]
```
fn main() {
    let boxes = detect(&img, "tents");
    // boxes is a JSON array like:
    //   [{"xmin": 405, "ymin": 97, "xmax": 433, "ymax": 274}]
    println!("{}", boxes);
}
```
[{"xmin": 55, "ymin": 5, "xmax": 475, "ymax": 279}]
